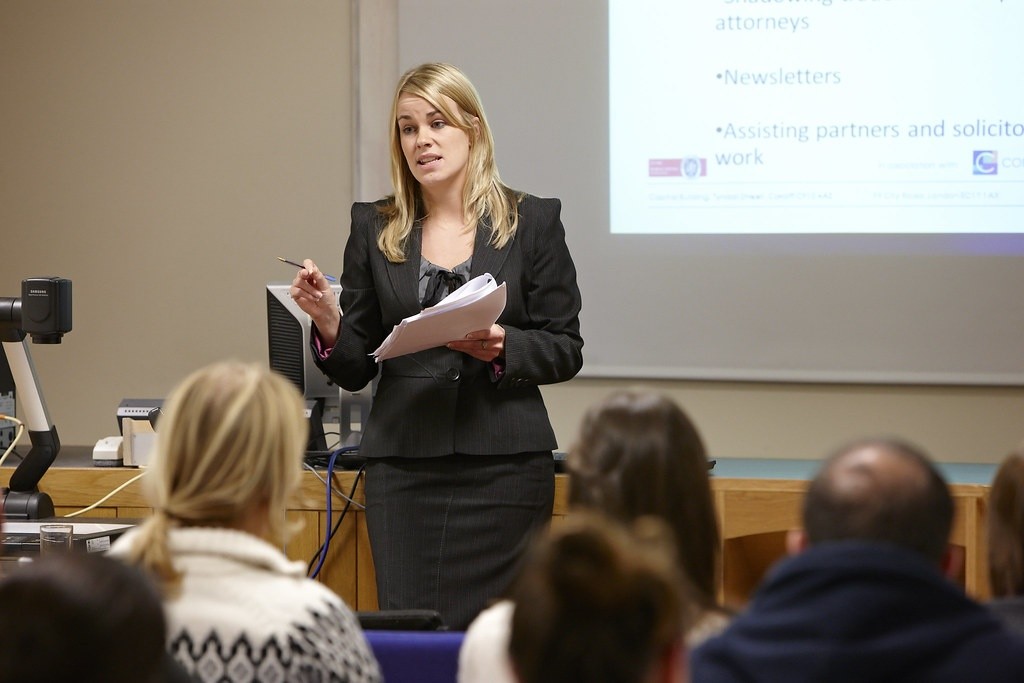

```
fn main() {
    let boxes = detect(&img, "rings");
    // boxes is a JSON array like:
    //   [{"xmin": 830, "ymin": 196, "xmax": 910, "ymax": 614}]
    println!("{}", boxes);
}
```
[{"xmin": 482, "ymin": 340, "xmax": 487, "ymax": 350}]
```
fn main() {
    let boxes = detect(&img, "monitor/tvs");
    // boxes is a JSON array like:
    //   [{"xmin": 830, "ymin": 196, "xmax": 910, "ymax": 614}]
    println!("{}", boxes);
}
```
[{"xmin": 265, "ymin": 286, "xmax": 383, "ymax": 459}]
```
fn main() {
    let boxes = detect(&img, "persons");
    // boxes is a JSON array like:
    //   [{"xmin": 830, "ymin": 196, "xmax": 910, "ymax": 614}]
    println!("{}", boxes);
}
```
[
  {"xmin": 290, "ymin": 62, "xmax": 585, "ymax": 631},
  {"xmin": 457, "ymin": 390, "xmax": 1024, "ymax": 683},
  {"xmin": 0, "ymin": 550, "xmax": 191, "ymax": 683},
  {"xmin": 103, "ymin": 360, "xmax": 384, "ymax": 683}
]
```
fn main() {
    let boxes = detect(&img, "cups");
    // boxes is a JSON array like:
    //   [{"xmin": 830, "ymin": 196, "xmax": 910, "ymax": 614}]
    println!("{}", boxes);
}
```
[{"xmin": 40, "ymin": 525, "xmax": 73, "ymax": 557}]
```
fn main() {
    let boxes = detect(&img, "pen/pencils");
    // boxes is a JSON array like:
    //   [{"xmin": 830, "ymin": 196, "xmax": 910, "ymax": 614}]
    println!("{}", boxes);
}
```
[{"xmin": 277, "ymin": 257, "xmax": 336, "ymax": 282}]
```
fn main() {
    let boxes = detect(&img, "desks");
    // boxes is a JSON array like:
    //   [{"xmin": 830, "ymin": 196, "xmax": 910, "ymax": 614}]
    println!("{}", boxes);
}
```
[{"xmin": 0, "ymin": 466, "xmax": 996, "ymax": 621}]
